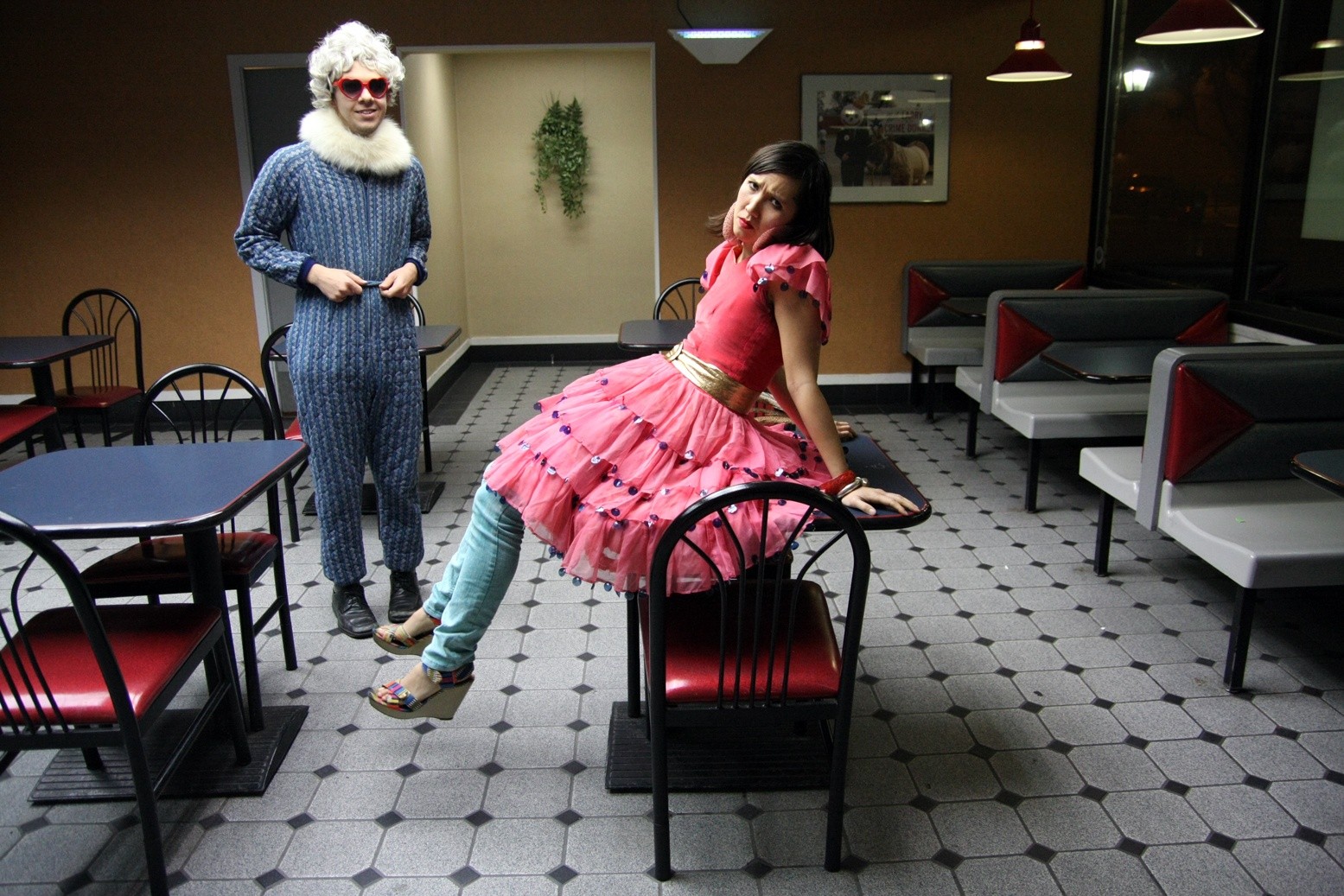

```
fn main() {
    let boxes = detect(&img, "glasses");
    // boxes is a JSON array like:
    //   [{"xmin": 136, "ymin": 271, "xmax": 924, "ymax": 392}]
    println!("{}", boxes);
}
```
[{"xmin": 333, "ymin": 76, "xmax": 392, "ymax": 100}]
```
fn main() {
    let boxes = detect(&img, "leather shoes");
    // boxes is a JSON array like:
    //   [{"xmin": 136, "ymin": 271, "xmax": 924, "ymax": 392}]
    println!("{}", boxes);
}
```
[
  {"xmin": 330, "ymin": 584, "xmax": 379, "ymax": 638},
  {"xmin": 387, "ymin": 570, "xmax": 423, "ymax": 624}
]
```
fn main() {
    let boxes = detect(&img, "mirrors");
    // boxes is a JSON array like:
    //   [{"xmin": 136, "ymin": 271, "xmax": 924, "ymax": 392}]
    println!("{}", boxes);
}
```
[{"xmin": 1080, "ymin": 0, "xmax": 1289, "ymax": 323}]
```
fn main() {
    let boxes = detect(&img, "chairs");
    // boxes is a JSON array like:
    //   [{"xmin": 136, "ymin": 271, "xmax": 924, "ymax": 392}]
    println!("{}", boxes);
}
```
[
  {"xmin": 79, "ymin": 361, "xmax": 297, "ymax": 732},
  {"xmin": 1, "ymin": 512, "xmax": 252, "ymax": 896},
  {"xmin": 407, "ymin": 291, "xmax": 432, "ymax": 468},
  {"xmin": 3, "ymin": 404, "xmax": 58, "ymax": 473},
  {"xmin": 19, "ymin": 287, "xmax": 147, "ymax": 460},
  {"xmin": 654, "ymin": 278, "xmax": 707, "ymax": 322},
  {"xmin": 259, "ymin": 319, "xmax": 381, "ymax": 541},
  {"xmin": 627, "ymin": 482, "xmax": 869, "ymax": 883}
]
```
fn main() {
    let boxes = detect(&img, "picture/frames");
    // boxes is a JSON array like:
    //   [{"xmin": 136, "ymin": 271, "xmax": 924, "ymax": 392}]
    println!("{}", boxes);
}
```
[{"xmin": 801, "ymin": 72, "xmax": 952, "ymax": 205}]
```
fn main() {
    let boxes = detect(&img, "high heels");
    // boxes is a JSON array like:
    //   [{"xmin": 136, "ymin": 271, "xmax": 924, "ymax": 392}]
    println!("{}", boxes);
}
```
[
  {"xmin": 372, "ymin": 622, "xmax": 434, "ymax": 656},
  {"xmin": 366, "ymin": 664, "xmax": 474, "ymax": 720}
]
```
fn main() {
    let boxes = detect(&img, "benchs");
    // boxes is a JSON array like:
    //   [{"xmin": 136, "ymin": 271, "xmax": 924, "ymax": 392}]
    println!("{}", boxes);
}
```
[
  {"xmin": 954, "ymin": 290, "xmax": 1235, "ymax": 515},
  {"xmin": 897, "ymin": 258, "xmax": 1103, "ymax": 425},
  {"xmin": 1076, "ymin": 344, "xmax": 1344, "ymax": 695}
]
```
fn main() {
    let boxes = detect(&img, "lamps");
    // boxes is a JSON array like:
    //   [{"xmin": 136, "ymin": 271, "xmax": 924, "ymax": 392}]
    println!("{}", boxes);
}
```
[
  {"xmin": 665, "ymin": 26, "xmax": 775, "ymax": 64},
  {"xmin": 1120, "ymin": 53, "xmax": 1153, "ymax": 92},
  {"xmin": 1279, "ymin": 15, "xmax": 1344, "ymax": 80},
  {"xmin": 986, "ymin": 0, "xmax": 1073, "ymax": 83},
  {"xmin": 1132, "ymin": 0, "xmax": 1265, "ymax": 46}
]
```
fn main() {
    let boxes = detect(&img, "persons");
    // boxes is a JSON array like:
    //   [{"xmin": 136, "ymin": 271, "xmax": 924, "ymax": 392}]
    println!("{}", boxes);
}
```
[
  {"xmin": 235, "ymin": 12, "xmax": 434, "ymax": 640},
  {"xmin": 357, "ymin": 133, "xmax": 927, "ymax": 723}
]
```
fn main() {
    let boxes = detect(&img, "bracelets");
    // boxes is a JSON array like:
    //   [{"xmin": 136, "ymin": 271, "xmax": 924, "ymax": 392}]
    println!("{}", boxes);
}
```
[{"xmin": 833, "ymin": 475, "xmax": 869, "ymax": 499}]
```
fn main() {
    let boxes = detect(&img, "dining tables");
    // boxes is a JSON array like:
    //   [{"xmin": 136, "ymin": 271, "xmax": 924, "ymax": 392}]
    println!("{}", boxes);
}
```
[
  {"xmin": 1039, "ymin": 341, "xmax": 1293, "ymax": 385},
  {"xmin": 1, "ymin": 438, "xmax": 314, "ymax": 809},
  {"xmin": 940, "ymin": 296, "xmax": 987, "ymax": 319},
  {"xmin": 1286, "ymin": 446, "xmax": 1344, "ymax": 497},
  {"xmin": 616, "ymin": 320, "xmax": 697, "ymax": 352},
  {"xmin": 261, "ymin": 326, "xmax": 465, "ymax": 513},
  {"xmin": 605, "ymin": 435, "xmax": 930, "ymax": 791},
  {"xmin": 0, "ymin": 333, "xmax": 115, "ymax": 452}
]
